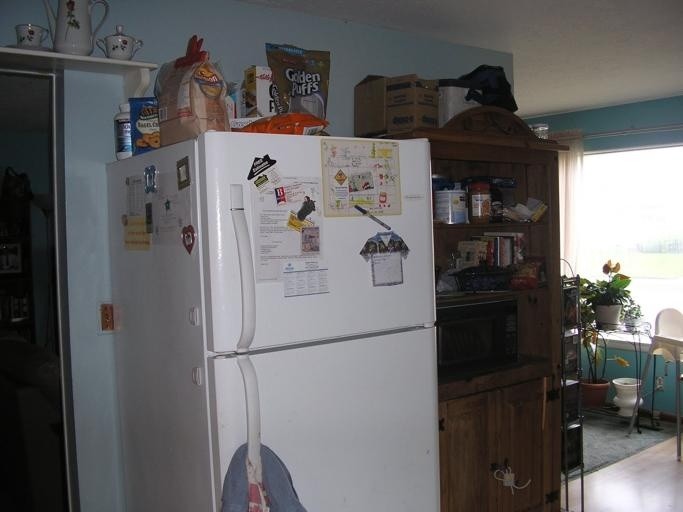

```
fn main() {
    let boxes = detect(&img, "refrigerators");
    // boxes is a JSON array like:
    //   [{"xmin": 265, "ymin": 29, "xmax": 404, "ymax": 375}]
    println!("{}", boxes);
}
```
[{"xmin": 103, "ymin": 131, "xmax": 442, "ymax": 512}]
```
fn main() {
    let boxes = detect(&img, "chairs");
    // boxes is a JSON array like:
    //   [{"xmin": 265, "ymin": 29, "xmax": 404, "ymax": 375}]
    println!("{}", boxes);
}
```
[{"xmin": 627, "ymin": 307, "xmax": 683, "ymax": 463}]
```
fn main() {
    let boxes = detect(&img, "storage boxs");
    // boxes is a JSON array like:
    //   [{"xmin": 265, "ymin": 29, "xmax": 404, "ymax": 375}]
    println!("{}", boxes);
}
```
[{"xmin": 353, "ymin": 73, "xmax": 439, "ymax": 135}]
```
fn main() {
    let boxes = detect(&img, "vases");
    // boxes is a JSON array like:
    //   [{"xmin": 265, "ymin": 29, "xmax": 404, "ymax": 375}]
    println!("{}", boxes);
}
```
[{"xmin": 611, "ymin": 377, "xmax": 644, "ymax": 417}]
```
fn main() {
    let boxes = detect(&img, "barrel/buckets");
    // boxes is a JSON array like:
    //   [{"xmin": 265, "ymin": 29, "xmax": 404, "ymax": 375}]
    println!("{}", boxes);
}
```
[{"xmin": 433, "ymin": 184, "xmax": 470, "ymax": 225}]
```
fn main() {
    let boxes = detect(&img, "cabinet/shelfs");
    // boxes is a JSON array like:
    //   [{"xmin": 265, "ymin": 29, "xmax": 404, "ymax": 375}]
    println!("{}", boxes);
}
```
[{"xmin": 431, "ymin": 139, "xmax": 574, "ymax": 508}]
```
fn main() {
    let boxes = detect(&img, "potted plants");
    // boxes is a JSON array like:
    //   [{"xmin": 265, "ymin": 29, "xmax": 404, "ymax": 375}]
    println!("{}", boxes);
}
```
[
  {"xmin": 578, "ymin": 304, "xmax": 629, "ymax": 408},
  {"xmin": 578, "ymin": 259, "xmax": 641, "ymax": 332}
]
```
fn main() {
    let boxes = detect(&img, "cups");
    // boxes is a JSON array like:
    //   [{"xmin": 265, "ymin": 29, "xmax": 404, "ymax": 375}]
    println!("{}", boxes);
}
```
[
  {"xmin": 95, "ymin": 24, "xmax": 144, "ymax": 61},
  {"xmin": 14, "ymin": 23, "xmax": 50, "ymax": 47}
]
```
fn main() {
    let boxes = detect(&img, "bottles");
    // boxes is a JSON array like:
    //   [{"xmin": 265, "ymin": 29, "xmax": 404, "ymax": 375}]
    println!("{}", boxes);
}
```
[{"xmin": 113, "ymin": 102, "xmax": 132, "ymax": 161}]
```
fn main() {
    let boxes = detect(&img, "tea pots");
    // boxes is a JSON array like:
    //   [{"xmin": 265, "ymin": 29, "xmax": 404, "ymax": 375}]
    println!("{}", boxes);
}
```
[{"xmin": 42, "ymin": 0, "xmax": 109, "ymax": 57}]
